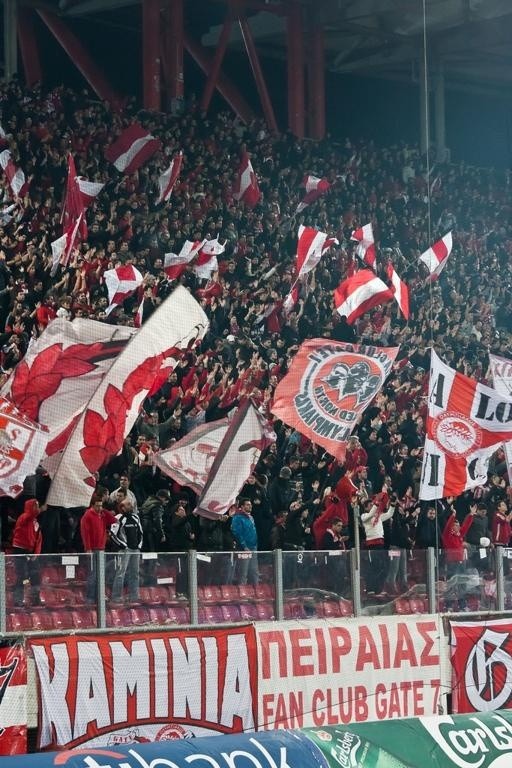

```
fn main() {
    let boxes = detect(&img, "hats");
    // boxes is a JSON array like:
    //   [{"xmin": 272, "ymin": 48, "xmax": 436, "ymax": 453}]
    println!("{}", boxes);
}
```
[
  {"xmin": 156, "ymin": 489, "xmax": 173, "ymax": 504},
  {"xmin": 355, "ymin": 465, "xmax": 369, "ymax": 473}
]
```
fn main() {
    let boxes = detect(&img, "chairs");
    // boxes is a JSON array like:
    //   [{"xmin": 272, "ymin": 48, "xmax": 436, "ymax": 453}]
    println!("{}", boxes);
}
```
[
  {"xmin": 396, "ymin": 597, "xmax": 510, "ymax": 615},
  {"xmin": 0, "ymin": 562, "xmax": 355, "ymax": 633}
]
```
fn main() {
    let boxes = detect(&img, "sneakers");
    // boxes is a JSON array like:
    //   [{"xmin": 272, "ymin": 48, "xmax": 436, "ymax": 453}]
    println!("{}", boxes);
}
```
[
  {"xmin": 29, "ymin": 602, "xmax": 46, "ymax": 609},
  {"xmin": 110, "ymin": 600, "xmax": 125, "ymax": 607},
  {"xmin": 175, "ymin": 592, "xmax": 188, "ymax": 601},
  {"xmin": 128, "ymin": 598, "xmax": 147, "ymax": 606},
  {"xmin": 13, "ymin": 601, "xmax": 26, "ymax": 610}
]
